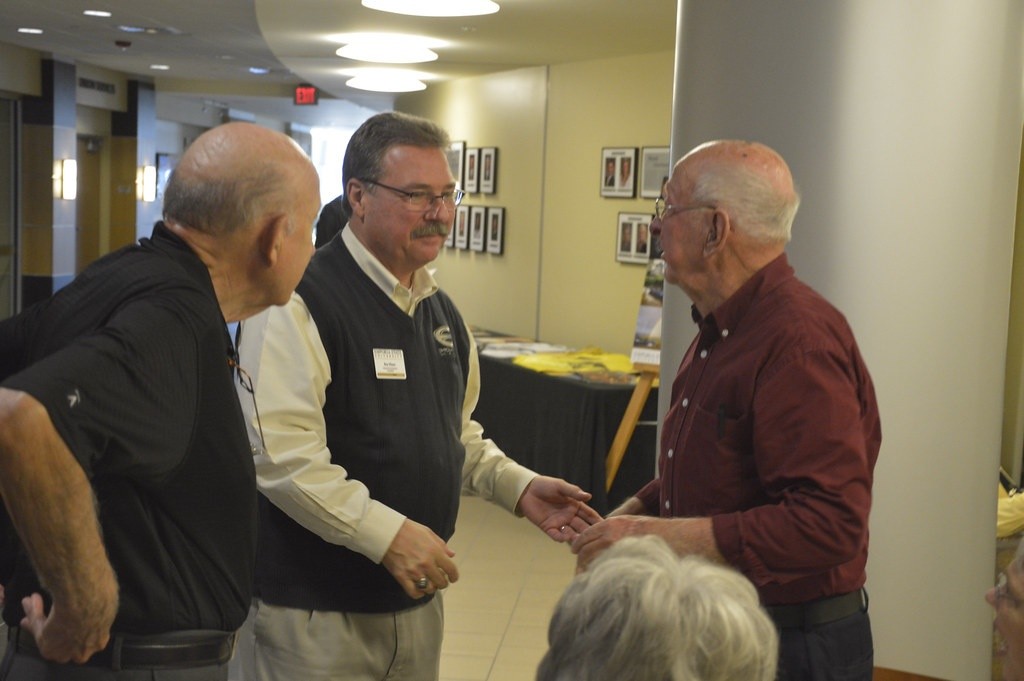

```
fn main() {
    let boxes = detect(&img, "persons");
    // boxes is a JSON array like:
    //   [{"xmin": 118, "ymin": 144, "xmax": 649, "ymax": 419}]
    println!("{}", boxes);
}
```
[
  {"xmin": 0, "ymin": 122, "xmax": 321, "ymax": 681},
  {"xmin": 619, "ymin": 158, "xmax": 630, "ymax": 189},
  {"xmin": 227, "ymin": 113, "xmax": 602, "ymax": 681},
  {"xmin": 621, "ymin": 222, "xmax": 632, "ymax": 252},
  {"xmin": 638, "ymin": 223, "xmax": 648, "ymax": 253},
  {"xmin": 605, "ymin": 159, "xmax": 614, "ymax": 185},
  {"xmin": 571, "ymin": 140, "xmax": 882, "ymax": 681},
  {"xmin": 984, "ymin": 540, "xmax": 1024, "ymax": 681},
  {"xmin": 536, "ymin": 535, "xmax": 780, "ymax": 681}
]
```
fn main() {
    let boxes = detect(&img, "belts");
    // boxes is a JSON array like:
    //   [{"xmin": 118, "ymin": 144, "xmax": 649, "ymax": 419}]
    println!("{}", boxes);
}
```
[
  {"xmin": 761, "ymin": 589, "xmax": 864, "ymax": 630},
  {"xmin": 7, "ymin": 626, "xmax": 233, "ymax": 669}
]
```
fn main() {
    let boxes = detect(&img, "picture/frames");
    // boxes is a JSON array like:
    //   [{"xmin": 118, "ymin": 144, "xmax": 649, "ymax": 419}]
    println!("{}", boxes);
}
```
[
  {"xmin": 601, "ymin": 148, "xmax": 640, "ymax": 199},
  {"xmin": 469, "ymin": 206, "xmax": 487, "ymax": 253},
  {"xmin": 465, "ymin": 149, "xmax": 480, "ymax": 194},
  {"xmin": 487, "ymin": 207, "xmax": 506, "ymax": 256},
  {"xmin": 443, "ymin": 208, "xmax": 455, "ymax": 248},
  {"xmin": 480, "ymin": 147, "xmax": 497, "ymax": 193},
  {"xmin": 444, "ymin": 141, "xmax": 464, "ymax": 196},
  {"xmin": 639, "ymin": 147, "xmax": 670, "ymax": 199},
  {"xmin": 455, "ymin": 206, "xmax": 470, "ymax": 250},
  {"xmin": 615, "ymin": 212, "xmax": 653, "ymax": 264}
]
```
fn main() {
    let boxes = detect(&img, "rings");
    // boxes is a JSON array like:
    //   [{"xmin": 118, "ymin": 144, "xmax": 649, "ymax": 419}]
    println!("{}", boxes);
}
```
[
  {"xmin": 418, "ymin": 576, "xmax": 430, "ymax": 590},
  {"xmin": 559, "ymin": 525, "xmax": 567, "ymax": 531}
]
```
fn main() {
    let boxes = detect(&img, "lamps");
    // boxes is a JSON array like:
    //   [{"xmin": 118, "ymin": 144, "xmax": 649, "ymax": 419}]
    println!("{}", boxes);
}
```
[
  {"xmin": 63, "ymin": 159, "xmax": 77, "ymax": 200},
  {"xmin": 336, "ymin": 43, "xmax": 438, "ymax": 64},
  {"xmin": 345, "ymin": 72, "xmax": 428, "ymax": 92},
  {"xmin": 143, "ymin": 166, "xmax": 156, "ymax": 201},
  {"xmin": 362, "ymin": 0, "xmax": 500, "ymax": 17}
]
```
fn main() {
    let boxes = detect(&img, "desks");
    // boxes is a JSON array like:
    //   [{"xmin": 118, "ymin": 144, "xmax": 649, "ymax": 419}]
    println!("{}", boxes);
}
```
[{"xmin": 467, "ymin": 324, "xmax": 659, "ymax": 518}]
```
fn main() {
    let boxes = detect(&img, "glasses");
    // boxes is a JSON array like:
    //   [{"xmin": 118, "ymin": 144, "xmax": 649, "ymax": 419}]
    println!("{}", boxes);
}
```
[
  {"xmin": 655, "ymin": 196, "xmax": 733, "ymax": 229},
  {"xmin": 358, "ymin": 178, "xmax": 466, "ymax": 210}
]
